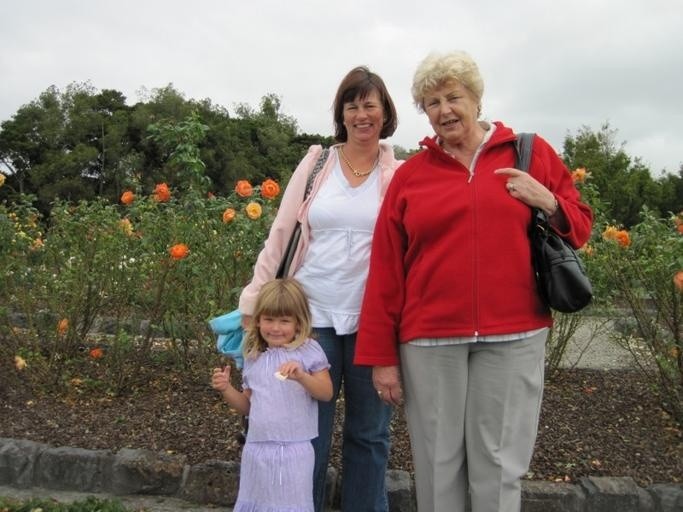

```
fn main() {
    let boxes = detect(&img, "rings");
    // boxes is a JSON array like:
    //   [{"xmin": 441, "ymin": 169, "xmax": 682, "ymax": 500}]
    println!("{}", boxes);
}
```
[
  {"xmin": 510, "ymin": 182, "xmax": 514, "ymax": 191},
  {"xmin": 376, "ymin": 391, "xmax": 382, "ymax": 395}
]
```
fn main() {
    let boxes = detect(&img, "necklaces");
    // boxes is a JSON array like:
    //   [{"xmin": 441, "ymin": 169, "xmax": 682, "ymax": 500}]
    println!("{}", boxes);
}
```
[{"xmin": 340, "ymin": 142, "xmax": 379, "ymax": 176}]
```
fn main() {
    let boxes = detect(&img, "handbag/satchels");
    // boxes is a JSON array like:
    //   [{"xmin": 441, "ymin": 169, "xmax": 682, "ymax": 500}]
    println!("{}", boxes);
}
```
[{"xmin": 534, "ymin": 208, "xmax": 592, "ymax": 313}]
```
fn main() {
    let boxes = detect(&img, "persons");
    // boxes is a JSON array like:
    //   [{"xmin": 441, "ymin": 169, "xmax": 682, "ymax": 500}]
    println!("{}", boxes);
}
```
[
  {"xmin": 353, "ymin": 50, "xmax": 592, "ymax": 512},
  {"xmin": 212, "ymin": 279, "xmax": 335, "ymax": 512},
  {"xmin": 238, "ymin": 68, "xmax": 406, "ymax": 512}
]
